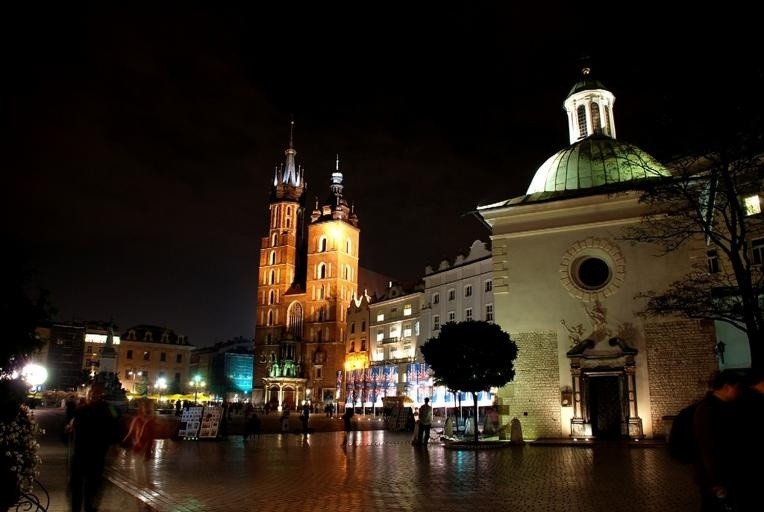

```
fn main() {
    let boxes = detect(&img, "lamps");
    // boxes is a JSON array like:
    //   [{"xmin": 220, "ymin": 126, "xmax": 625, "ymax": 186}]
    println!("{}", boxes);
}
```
[{"xmin": 714, "ymin": 341, "xmax": 726, "ymax": 364}]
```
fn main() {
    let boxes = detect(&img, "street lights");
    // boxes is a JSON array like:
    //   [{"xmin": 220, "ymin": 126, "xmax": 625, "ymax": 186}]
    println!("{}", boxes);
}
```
[
  {"xmin": 188, "ymin": 375, "xmax": 207, "ymax": 404},
  {"xmin": 128, "ymin": 371, "xmax": 143, "ymax": 397},
  {"xmin": 153, "ymin": 376, "xmax": 168, "ymax": 399}
]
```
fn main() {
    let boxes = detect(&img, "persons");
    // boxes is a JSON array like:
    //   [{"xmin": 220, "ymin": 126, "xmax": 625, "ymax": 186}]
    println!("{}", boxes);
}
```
[
  {"xmin": 122, "ymin": 395, "xmax": 157, "ymax": 490},
  {"xmin": 411, "ymin": 397, "xmax": 433, "ymax": 448},
  {"xmin": 618, "ymin": 322, "xmax": 638, "ymax": 348},
  {"xmin": 735, "ymin": 361, "xmax": 764, "ymax": 512},
  {"xmin": 165, "ymin": 399, "xmax": 253, "ymax": 416},
  {"xmin": 581, "ymin": 298, "xmax": 609, "ymax": 332},
  {"xmin": 560, "ymin": 321, "xmax": 583, "ymax": 348},
  {"xmin": 692, "ymin": 368, "xmax": 744, "ymax": 512},
  {"xmin": 263, "ymin": 401, "xmax": 355, "ymax": 432},
  {"xmin": 59, "ymin": 384, "xmax": 122, "ymax": 511},
  {"xmin": 66, "ymin": 396, "xmax": 86, "ymax": 420}
]
